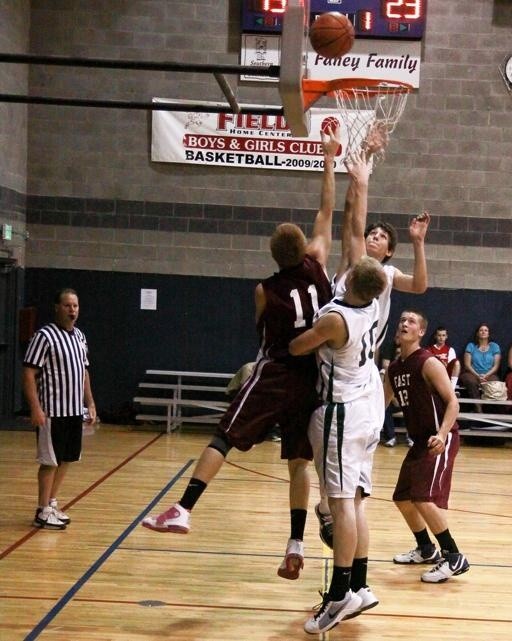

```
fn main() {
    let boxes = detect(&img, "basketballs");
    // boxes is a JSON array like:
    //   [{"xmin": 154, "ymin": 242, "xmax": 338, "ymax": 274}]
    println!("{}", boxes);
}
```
[{"xmin": 309, "ymin": 12, "xmax": 355, "ymax": 58}]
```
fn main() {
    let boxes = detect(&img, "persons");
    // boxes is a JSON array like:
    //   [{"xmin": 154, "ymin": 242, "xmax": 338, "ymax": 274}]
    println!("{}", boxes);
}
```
[
  {"xmin": 142, "ymin": 120, "xmax": 340, "ymax": 580},
  {"xmin": 505, "ymin": 346, "xmax": 512, "ymax": 400},
  {"xmin": 290, "ymin": 148, "xmax": 386, "ymax": 635},
  {"xmin": 461, "ymin": 323, "xmax": 503, "ymax": 412},
  {"xmin": 309, "ymin": 123, "xmax": 431, "ymax": 550},
  {"xmin": 380, "ymin": 330, "xmax": 414, "ymax": 446},
  {"xmin": 23, "ymin": 288, "xmax": 95, "ymax": 529},
  {"xmin": 383, "ymin": 307, "xmax": 470, "ymax": 584},
  {"xmin": 426, "ymin": 327, "xmax": 461, "ymax": 393}
]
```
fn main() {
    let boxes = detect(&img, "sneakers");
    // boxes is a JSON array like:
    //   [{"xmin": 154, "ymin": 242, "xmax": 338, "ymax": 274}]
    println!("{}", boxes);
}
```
[
  {"xmin": 384, "ymin": 431, "xmax": 415, "ymax": 448},
  {"xmin": 420, "ymin": 550, "xmax": 470, "ymax": 584},
  {"xmin": 277, "ymin": 537, "xmax": 305, "ymax": 580},
  {"xmin": 141, "ymin": 500, "xmax": 192, "ymax": 535},
  {"xmin": 314, "ymin": 502, "xmax": 334, "ymax": 550},
  {"xmin": 303, "ymin": 583, "xmax": 379, "ymax": 635},
  {"xmin": 392, "ymin": 543, "xmax": 441, "ymax": 565},
  {"xmin": 31, "ymin": 497, "xmax": 71, "ymax": 531}
]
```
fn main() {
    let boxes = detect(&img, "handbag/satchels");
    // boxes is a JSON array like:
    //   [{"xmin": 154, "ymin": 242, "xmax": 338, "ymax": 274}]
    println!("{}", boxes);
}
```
[{"xmin": 479, "ymin": 379, "xmax": 509, "ymax": 403}]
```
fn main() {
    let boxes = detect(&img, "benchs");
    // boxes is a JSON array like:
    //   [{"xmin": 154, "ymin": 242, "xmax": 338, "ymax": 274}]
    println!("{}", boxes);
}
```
[
  {"xmin": 455, "ymin": 385, "xmax": 512, "ymax": 442},
  {"xmin": 382, "ymin": 410, "xmax": 408, "ymax": 433},
  {"xmin": 133, "ymin": 370, "xmax": 237, "ymax": 434}
]
